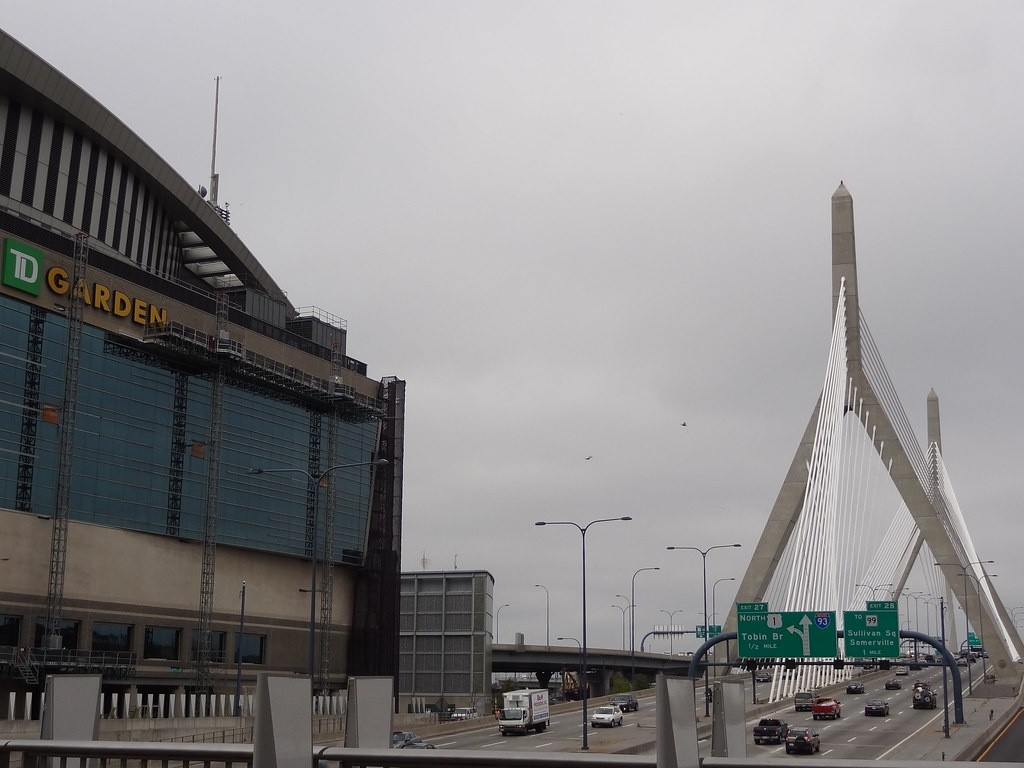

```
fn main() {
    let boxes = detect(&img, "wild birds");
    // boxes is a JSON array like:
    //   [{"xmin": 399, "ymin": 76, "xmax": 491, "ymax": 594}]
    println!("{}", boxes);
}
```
[
  {"xmin": 584, "ymin": 456, "xmax": 592, "ymax": 461},
  {"xmin": 681, "ymin": 421, "xmax": 687, "ymax": 427}
]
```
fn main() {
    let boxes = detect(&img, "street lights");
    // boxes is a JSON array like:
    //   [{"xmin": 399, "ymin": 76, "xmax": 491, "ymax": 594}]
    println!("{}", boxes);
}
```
[
  {"xmin": 535, "ymin": 584, "xmax": 549, "ymax": 647},
  {"xmin": 667, "ymin": 544, "xmax": 742, "ymax": 717},
  {"xmin": 957, "ymin": 573, "xmax": 998, "ymax": 687},
  {"xmin": 873, "ymin": 586, "xmax": 943, "ymax": 661},
  {"xmin": 713, "ymin": 577, "xmax": 735, "ymax": 678},
  {"xmin": 615, "ymin": 594, "xmax": 631, "ymax": 651},
  {"xmin": 1004, "ymin": 606, "xmax": 1024, "ymax": 631},
  {"xmin": 855, "ymin": 583, "xmax": 892, "ymax": 601},
  {"xmin": 534, "ymin": 516, "xmax": 633, "ymax": 751},
  {"xmin": 659, "ymin": 609, "xmax": 682, "ymax": 657},
  {"xmin": 632, "ymin": 567, "xmax": 660, "ymax": 678},
  {"xmin": 698, "ymin": 612, "xmax": 719, "ymax": 655},
  {"xmin": 611, "ymin": 604, "xmax": 636, "ymax": 651},
  {"xmin": 259, "ymin": 458, "xmax": 390, "ymax": 681},
  {"xmin": 933, "ymin": 560, "xmax": 994, "ymax": 696},
  {"xmin": 496, "ymin": 604, "xmax": 510, "ymax": 645},
  {"xmin": 557, "ymin": 637, "xmax": 582, "ymax": 701}
]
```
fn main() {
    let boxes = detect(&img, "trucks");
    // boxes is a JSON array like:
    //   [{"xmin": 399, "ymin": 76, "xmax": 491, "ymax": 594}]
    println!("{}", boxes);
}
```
[{"xmin": 497, "ymin": 689, "xmax": 551, "ymax": 737}]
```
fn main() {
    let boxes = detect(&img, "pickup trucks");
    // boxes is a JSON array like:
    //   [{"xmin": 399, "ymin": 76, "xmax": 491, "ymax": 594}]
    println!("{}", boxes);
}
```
[
  {"xmin": 753, "ymin": 718, "xmax": 789, "ymax": 745},
  {"xmin": 811, "ymin": 697, "xmax": 841, "ymax": 721}
]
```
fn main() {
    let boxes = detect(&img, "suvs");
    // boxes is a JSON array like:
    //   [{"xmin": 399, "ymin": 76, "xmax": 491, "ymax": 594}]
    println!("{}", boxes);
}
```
[
  {"xmin": 591, "ymin": 707, "xmax": 622, "ymax": 728},
  {"xmin": 450, "ymin": 707, "xmax": 477, "ymax": 721},
  {"xmin": 786, "ymin": 727, "xmax": 820, "ymax": 755},
  {"xmin": 913, "ymin": 682, "xmax": 938, "ymax": 710},
  {"xmin": 610, "ymin": 694, "xmax": 639, "ymax": 713},
  {"xmin": 794, "ymin": 693, "xmax": 820, "ymax": 712}
]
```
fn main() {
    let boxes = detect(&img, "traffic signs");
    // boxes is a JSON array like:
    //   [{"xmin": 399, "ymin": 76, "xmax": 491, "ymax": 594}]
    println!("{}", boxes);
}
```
[{"xmin": 738, "ymin": 611, "xmax": 837, "ymax": 658}]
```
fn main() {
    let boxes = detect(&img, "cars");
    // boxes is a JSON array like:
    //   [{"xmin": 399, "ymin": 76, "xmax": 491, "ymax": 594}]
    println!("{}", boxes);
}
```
[
  {"xmin": 846, "ymin": 681, "xmax": 865, "ymax": 694},
  {"xmin": 865, "ymin": 699, "xmax": 889, "ymax": 717},
  {"xmin": 895, "ymin": 648, "xmax": 989, "ymax": 676},
  {"xmin": 756, "ymin": 672, "xmax": 773, "ymax": 683},
  {"xmin": 885, "ymin": 678, "xmax": 902, "ymax": 690},
  {"xmin": 393, "ymin": 730, "xmax": 422, "ymax": 750},
  {"xmin": 403, "ymin": 742, "xmax": 437, "ymax": 749}
]
